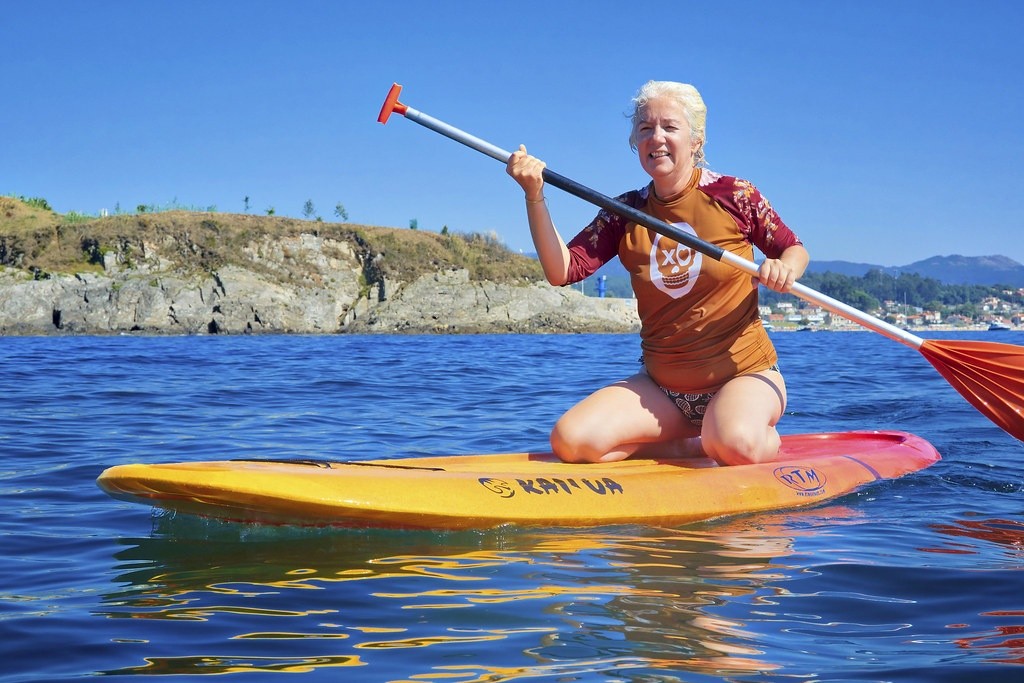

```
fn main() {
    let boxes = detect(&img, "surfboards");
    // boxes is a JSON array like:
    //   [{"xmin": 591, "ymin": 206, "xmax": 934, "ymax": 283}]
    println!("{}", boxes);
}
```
[{"xmin": 94, "ymin": 430, "xmax": 943, "ymax": 527}]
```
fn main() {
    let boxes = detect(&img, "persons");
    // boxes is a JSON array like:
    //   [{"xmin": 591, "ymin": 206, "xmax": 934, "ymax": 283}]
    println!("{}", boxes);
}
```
[{"xmin": 507, "ymin": 80, "xmax": 810, "ymax": 466}]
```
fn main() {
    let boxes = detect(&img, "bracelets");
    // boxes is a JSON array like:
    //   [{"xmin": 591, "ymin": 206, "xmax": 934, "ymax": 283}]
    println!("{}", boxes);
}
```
[{"xmin": 524, "ymin": 194, "xmax": 545, "ymax": 204}]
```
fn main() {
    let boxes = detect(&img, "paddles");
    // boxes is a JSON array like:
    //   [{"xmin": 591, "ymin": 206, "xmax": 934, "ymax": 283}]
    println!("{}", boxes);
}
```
[{"xmin": 378, "ymin": 76, "xmax": 1024, "ymax": 422}]
